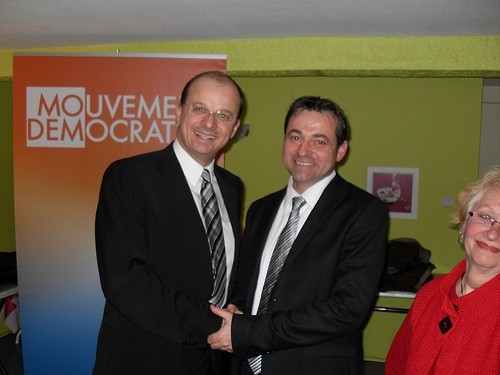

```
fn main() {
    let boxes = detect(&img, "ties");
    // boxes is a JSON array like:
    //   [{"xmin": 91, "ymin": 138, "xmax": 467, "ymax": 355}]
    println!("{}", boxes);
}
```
[
  {"xmin": 200, "ymin": 168, "xmax": 227, "ymax": 309},
  {"xmin": 247, "ymin": 196, "xmax": 307, "ymax": 375}
]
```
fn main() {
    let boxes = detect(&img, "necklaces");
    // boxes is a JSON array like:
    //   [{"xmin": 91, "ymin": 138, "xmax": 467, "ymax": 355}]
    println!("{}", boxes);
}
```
[{"xmin": 460, "ymin": 272, "xmax": 464, "ymax": 296}]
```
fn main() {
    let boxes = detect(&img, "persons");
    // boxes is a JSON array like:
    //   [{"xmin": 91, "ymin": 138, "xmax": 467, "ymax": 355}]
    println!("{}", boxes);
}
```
[
  {"xmin": 384, "ymin": 167, "xmax": 500, "ymax": 375},
  {"xmin": 207, "ymin": 96, "xmax": 390, "ymax": 375},
  {"xmin": 93, "ymin": 70, "xmax": 246, "ymax": 375}
]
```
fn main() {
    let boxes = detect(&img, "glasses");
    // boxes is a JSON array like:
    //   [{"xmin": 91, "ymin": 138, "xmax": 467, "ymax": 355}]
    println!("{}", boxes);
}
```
[
  {"xmin": 468, "ymin": 209, "xmax": 500, "ymax": 225},
  {"xmin": 179, "ymin": 102, "xmax": 238, "ymax": 121}
]
